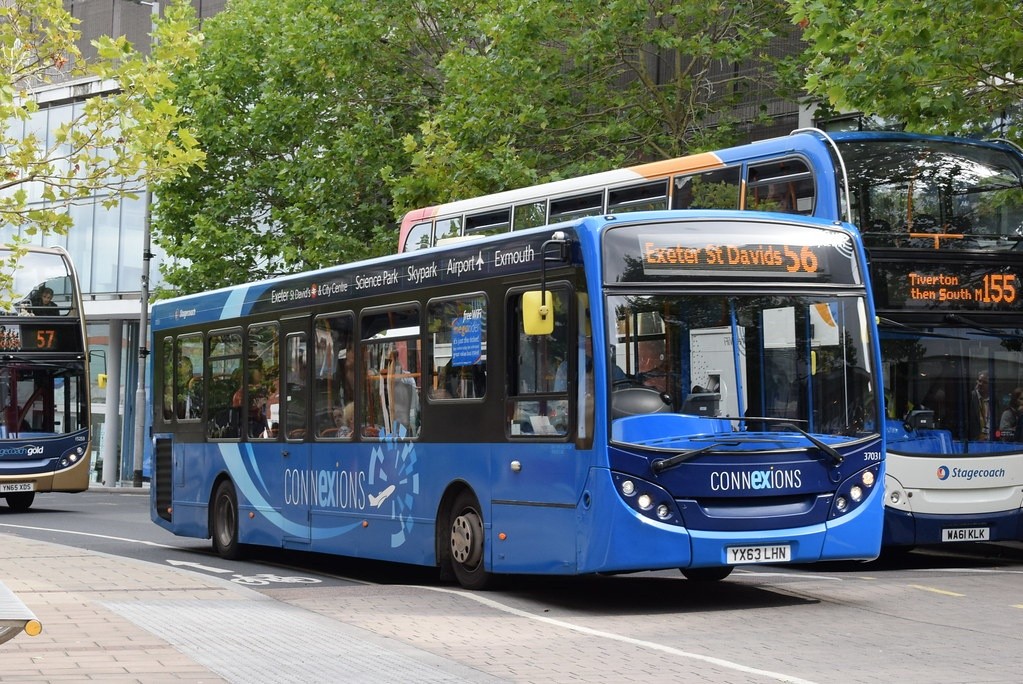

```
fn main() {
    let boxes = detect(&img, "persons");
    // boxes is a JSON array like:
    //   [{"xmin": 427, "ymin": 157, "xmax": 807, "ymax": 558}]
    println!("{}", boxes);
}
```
[
  {"xmin": 958, "ymin": 369, "xmax": 995, "ymax": 441},
  {"xmin": 17, "ymin": 405, "xmax": 33, "ymax": 432},
  {"xmin": 175, "ymin": 337, "xmax": 631, "ymax": 455},
  {"xmin": 865, "ymin": 368, "xmax": 916, "ymax": 430},
  {"xmin": 25, "ymin": 288, "xmax": 60, "ymax": 316},
  {"xmin": 999, "ymin": 388, "xmax": 1023, "ymax": 443}
]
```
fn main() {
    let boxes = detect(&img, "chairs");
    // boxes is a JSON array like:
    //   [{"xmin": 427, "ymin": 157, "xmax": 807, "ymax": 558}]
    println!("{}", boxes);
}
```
[
  {"xmin": 6, "ymin": 406, "xmax": 32, "ymax": 432},
  {"xmin": 185, "ymin": 375, "xmax": 380, "ymax": 438},
  {"xmin": 940, "ymin": 217, "xmax": 981, "ymax": 248},
  {"xmin": 863, "ymin": 219, "xmax": 897, "ymax": 248},
  {"xmin": 901, "ymin": 214, "xmax": 947, "ymax": 248}
]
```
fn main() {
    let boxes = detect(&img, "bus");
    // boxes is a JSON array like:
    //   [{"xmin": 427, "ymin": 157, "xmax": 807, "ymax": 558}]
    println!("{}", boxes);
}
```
[
  {"xmin": 398, "ymin": 127, "xmax": 1023, "ymax": 561},
  {"xmin": 150, "ymin": 208, "xmax": 887, "ymax": 590},
  {"xmin": 0, "ymin": 245, "xmax": 107, "ymax": 512}
]
